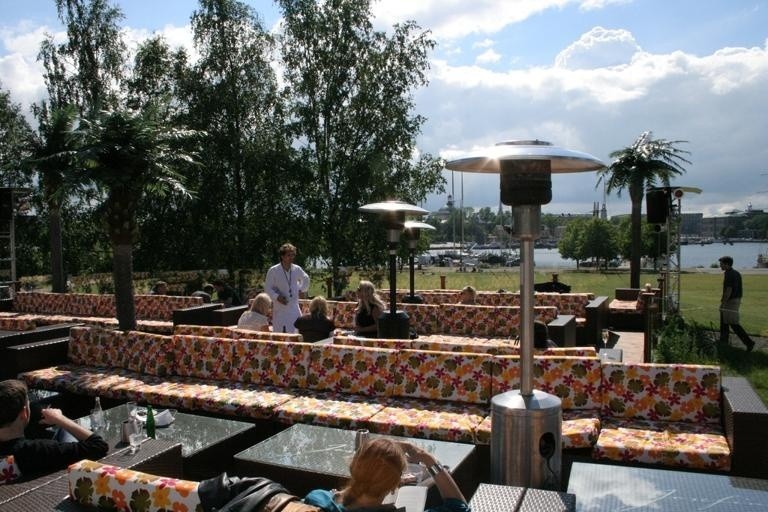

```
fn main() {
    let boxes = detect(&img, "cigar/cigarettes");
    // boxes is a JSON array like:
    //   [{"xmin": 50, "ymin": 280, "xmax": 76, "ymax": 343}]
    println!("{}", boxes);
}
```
[{"xmin": 40, "ymin": 403, "xmax": 51, "ymax": 418}]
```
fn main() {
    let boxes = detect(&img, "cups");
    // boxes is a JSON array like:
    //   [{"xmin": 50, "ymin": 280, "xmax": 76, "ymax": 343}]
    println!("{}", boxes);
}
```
[
  {"xmin": 119, "ymin": 400, "xmax": 142, "ymax": 451},
  {"xmin": 91, "ymin": 413, "xmax": 100, "ymax": 429}
]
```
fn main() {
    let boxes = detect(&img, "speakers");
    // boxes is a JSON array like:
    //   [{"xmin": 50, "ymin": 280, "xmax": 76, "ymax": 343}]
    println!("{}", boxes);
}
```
[{"xmin": 646, "ymin": 189, "xmax": 668, "ymax": 226}]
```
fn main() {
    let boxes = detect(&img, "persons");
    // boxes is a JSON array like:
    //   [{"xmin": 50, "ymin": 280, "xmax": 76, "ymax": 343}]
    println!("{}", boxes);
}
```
[
  {"xmin": 0, "ymin": 378, "xmax": 110, "ymax": 485},
  {"xmin": 266, "ymin": 243, "xmax": 311, "ymax": 332},
  {"xmin": 150, "ymin": 281, "xmax": 167, "ymax": 295},
  {"xmin": 293, "ymin": 296, "xmax": 334, "ymax": 343},
  {"xmin": 716, "ymin": 255, "xmax": 755, "ymax": 352},
  {"xmin": 213, "ymin": 279, "xmax": 243, "ymax": 307},
  {"xmin": 534, "ymin": 320, "xmax": 558, "ymax": 349},
  {"xmin": 457, "ymin": 286, "xmax": 482, "ymax": 305},
  {"xmin": 351, "ymin": 280, "xmax": 386, "ymax": 338},
  {"xmin": 304, "ymin": 437, "xmax": 473, "ymax": 512},
  {"xmin": 236, "ymin": 292, "xmax": 272, "ymax": 331},
  {"xmin": 191, "ymin": 285, "xmax": 214, "ymax": 304}
]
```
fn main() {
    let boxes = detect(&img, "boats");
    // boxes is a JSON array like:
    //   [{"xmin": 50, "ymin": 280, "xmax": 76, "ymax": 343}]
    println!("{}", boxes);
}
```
[
  {"xmin": 418, "ymin": 251, "xmax": 519, "ymax": 266},
  {"xmin": 578, "ymin": 259, "xmax": 621, "ymax": 270}
]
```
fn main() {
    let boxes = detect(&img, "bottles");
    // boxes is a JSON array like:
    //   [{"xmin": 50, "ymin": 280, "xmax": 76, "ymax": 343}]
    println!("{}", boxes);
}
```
[
  {"xmin": 356, "ymin": 429, "xmax": 369, "ymax": 452},
  {"xmin": 94, "ymin": 397, "xmax": 103, "ymax": 427},
  {"xmin": 146, "ymin": 404, "xmax": 155, "ymax": 440}
]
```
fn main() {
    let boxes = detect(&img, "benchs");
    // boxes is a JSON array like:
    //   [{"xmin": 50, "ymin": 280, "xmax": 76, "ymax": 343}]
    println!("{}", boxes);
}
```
[{"xmin": 0, "ymin": 292, "xmax": 767, "ymax": 472}]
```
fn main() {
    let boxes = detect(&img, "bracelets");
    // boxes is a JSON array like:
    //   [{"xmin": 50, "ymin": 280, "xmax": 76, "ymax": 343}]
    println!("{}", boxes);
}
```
[{"xmin": 426, "ymin": 460, "xmax": 444, "ymax": 477}]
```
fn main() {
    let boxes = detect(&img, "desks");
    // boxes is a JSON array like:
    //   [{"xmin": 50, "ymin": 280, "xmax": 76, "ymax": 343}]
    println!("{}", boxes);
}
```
[{"xmin": 0, "ymin": 387, "xmax": 768, "ymax": 512}]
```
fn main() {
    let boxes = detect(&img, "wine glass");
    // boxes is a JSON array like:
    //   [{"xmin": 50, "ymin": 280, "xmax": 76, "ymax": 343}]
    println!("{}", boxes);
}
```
[{"xmin": 602, "ymin": 329, "xmax": 609, "ymax": 355}]
